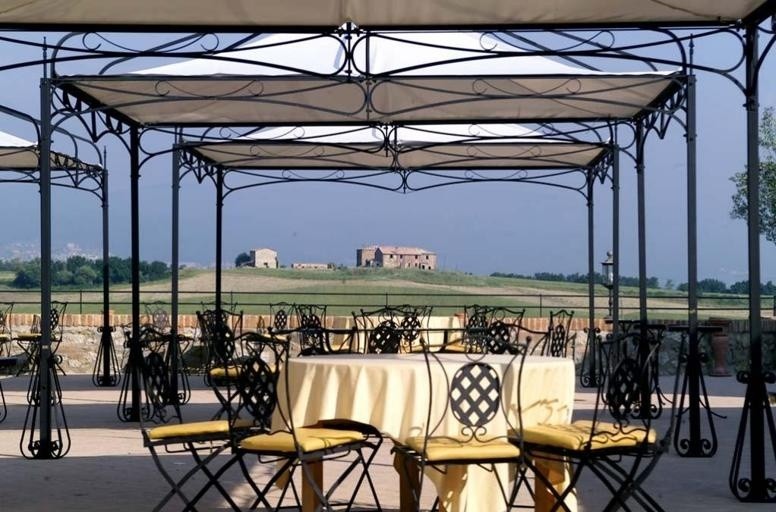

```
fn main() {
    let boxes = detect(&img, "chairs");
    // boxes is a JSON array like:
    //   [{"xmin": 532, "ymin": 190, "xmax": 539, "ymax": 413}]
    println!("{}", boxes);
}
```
[
  {"xmin": 117, "ymin": 300, "xmax": 686, "ymax": 512},
  {"xmin": 0, "ymin": 301, "xmax": 68, "ymax": 377}
]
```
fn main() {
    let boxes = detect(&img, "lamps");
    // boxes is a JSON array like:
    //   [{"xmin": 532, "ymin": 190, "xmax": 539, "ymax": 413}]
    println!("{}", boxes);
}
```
[{"xmin": 601, "ymin": 251, "xmax": 614, "ymax": 319}]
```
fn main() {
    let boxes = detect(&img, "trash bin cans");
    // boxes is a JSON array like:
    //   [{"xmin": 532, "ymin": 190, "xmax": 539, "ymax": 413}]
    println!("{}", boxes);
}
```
[{"xmin": 710, "ymin": 318, "xmax": 732, "ymax": 376}]
